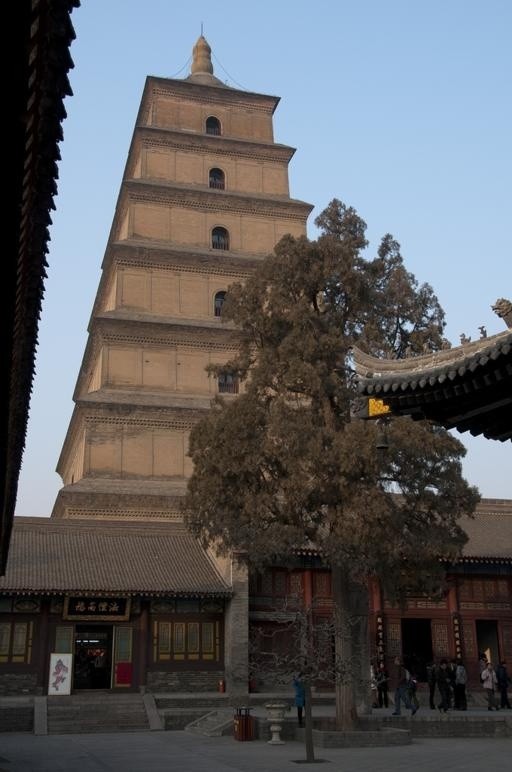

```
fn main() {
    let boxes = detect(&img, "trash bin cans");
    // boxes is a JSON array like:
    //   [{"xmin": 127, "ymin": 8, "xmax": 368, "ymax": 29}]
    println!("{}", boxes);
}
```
[{"xmin": 234, "ymin": 706, "xmax": 254, "ymax": 741}]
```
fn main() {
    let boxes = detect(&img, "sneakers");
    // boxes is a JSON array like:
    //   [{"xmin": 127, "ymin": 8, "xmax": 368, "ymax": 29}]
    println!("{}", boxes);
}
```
[
  {"xmin": 392, "ymin": 706, "xmax": 419, "ymax": 715},
  {"xmin": 488, "ymin": 704, "xmax": 511, "ymax": 711}
]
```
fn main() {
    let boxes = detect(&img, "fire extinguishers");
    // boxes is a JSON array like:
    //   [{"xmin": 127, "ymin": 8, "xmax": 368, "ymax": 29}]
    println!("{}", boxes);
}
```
[
  {"xmin": 217, "ymin": 678, "xmax": 224, "ymax": 693},
  {"xmin": 248, "ymin": 671, "xmax": 256, "ymax": 689}
]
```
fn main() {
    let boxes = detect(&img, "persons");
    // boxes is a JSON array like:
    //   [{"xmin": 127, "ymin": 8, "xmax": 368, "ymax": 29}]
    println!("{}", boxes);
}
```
[
  {"xmin": 370, "ymin": 655, "xmax": 511, "ymax": 715},
  {"xmin": 293, "ymin": 670, "xmax": 313, "ymax": 728}
]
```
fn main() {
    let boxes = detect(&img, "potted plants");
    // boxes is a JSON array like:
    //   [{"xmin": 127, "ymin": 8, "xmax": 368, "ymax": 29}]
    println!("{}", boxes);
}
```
[{"xmin": 264, "ymin": 697, "xmax": 292, "ymax": 744}]
